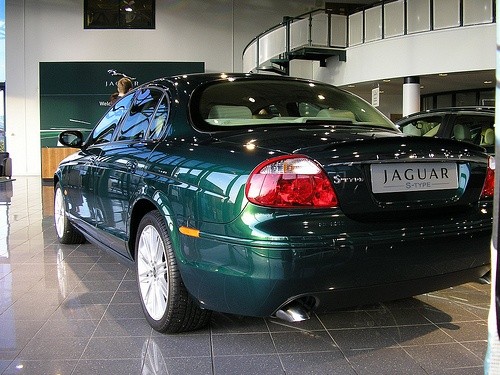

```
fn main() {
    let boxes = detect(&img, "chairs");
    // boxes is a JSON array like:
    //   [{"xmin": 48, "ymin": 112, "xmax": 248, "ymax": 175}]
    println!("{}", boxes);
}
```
[
  {"xmin": 252, "ymin": 114, "xmax": 271, "ymax": 119},
  {"xmin": 315, "ymin": 108, "xmax": 355, "ymax": 119},
  {"xmin": 209, "ymin": 105, "xmax": 253, "ymax": 120},
  {"xmin": 480, "ymin": 129, "xmax": 494, "ymax": 146}
]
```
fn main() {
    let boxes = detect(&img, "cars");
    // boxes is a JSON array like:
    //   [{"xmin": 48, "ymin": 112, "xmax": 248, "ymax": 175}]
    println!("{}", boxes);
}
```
[
  {"xmin": 391, "ymin": 104, "xmax": 496, "ymax": 157},
  {"xmin": 53, "ymin": 71, "xmax": 497, "ymax": 335}
]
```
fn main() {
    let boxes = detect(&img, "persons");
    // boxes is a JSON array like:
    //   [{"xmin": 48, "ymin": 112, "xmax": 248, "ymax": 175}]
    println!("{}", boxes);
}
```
[
  {"xmin": 117, "ymin": 78, "xmax": 133, "ymax": 101},
  {"xmin": 110, "ymin": 93, "xmax": 119, "ymax": 105}
]
